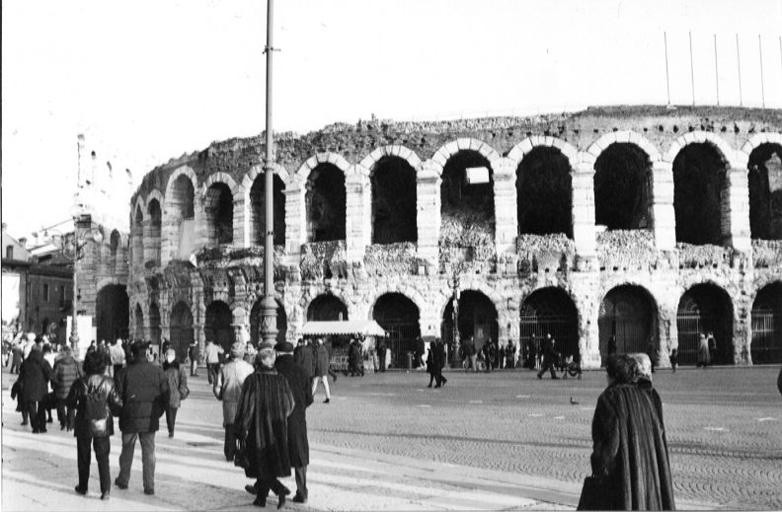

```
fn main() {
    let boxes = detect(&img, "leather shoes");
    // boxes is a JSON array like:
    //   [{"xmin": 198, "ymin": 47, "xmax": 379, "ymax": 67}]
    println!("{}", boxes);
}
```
[
  {"xmin": 20, "ymin": 418, "xmax": 174, "ymax": 500},
  {"xmin": 245, "ymin": 485, "xmax": 307, "ymax": 509}
]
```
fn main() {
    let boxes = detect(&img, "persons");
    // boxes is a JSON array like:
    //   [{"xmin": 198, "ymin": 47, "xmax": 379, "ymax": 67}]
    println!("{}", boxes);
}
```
[
  {"xmin": 377, "ymin": 341, "xmax": 387, "ymax": 371},
  {"xmin": 590, "ymin": 351, "xmax": 676, "ymax": 511},
  {"xmin": 404, "ymin": 329, "xmax": 560, "ymax": 388},
  {"xmin": 645, "ymin": 335, "xmax": 658, "ymax": 372},
  {"xmin": 353, "ymin": 340, "xmax": 365, "ymax": 376},
  {"xmin": 608, "ymin": 335, "xmax": 619, "ymax": 353},
  {"xmin": 627, "ymin": 353, "xmax": 664, "ymax": 420},
  {"xmin": 3, "ymin": 318, "xmax": 51, "ymax": 371},
  {"xmin": 65, "ymin": 338, "xmax": 189, "ymax": 495},
  {"xmin": 695, "ymin": 334, "xmax": 708, "ymax": 368},
  {"xmin": 342, "ymin": 338, "xmax": 364, "ymax": 376},
  {"xmin": 203, "ymin": 338, "xmax": 331, "ymax": 508},
  {"xmin": 9, "ymin": 344, "xmax": 83, "ymax": 433},
  {"xmin": 707, "ymin": 331, "xmax": 716, "ymax": 361},
  {"xmin": 669, "ymin": 349, "xmax": 680, "ymax": 370},
  {"xmin": 189, "ymin": 339, "xmax": 200, "ymax": 377}
]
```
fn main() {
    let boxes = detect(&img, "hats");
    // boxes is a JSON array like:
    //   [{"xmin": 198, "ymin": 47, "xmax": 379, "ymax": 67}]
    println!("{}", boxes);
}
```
[{"xmin": 274, "ymin": 342, "xmax": 293, "ymax": 352}]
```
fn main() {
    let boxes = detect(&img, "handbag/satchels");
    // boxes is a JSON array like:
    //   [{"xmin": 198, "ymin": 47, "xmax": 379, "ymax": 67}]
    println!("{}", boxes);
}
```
[{"xmin": 577, "ymin": 476, "xmax": 625, "ymax": 511}]
